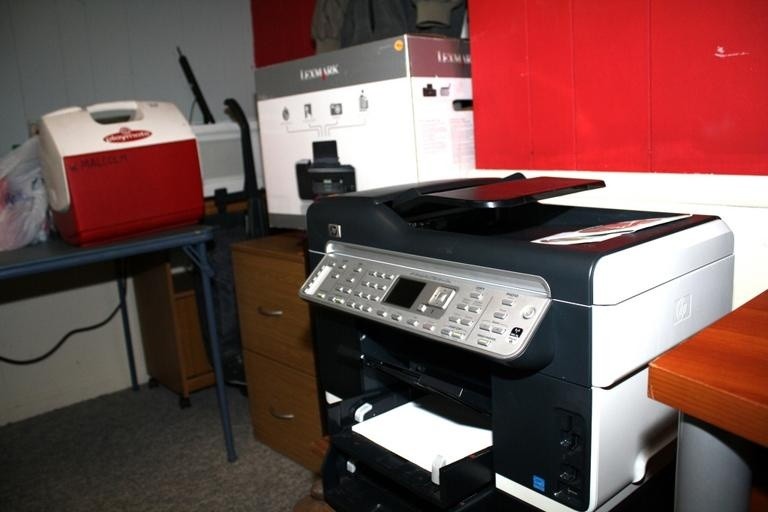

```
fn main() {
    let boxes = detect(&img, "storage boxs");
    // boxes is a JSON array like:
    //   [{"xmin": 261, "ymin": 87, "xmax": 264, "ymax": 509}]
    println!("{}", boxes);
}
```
[{"xmin": 254, "ymin": 35, "xmax": 490, "ymax": 232}]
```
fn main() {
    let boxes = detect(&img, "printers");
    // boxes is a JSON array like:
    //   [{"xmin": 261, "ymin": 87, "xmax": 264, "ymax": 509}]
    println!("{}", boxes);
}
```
[{"xmin": 298, "ymin": 173, "xmax": 734, "ymax": 512}]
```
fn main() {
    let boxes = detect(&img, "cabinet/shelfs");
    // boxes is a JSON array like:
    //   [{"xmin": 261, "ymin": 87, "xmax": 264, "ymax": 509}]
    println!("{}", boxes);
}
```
[
  {"xmin": 229, "ymin": 230, "xmax": 329, "ymax": 475},
  {"xmin": 468, "ymin": 0, "xmax": 768, "ymax": 176},
  {"xmin": 130, "ymin": 193, "xmax": 268, "ymax": 409}
]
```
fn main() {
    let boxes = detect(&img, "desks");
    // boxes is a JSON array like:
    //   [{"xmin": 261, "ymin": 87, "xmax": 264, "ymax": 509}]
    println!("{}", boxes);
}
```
[{"xmin": 0, "ymin": 221, "xmax": 237, "ymax": 465}]
